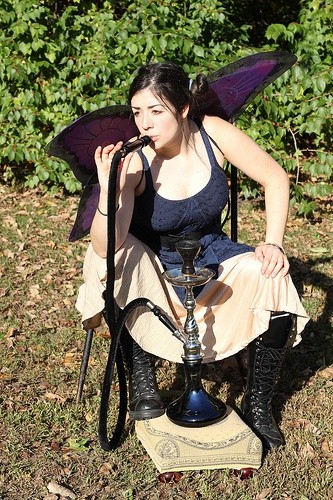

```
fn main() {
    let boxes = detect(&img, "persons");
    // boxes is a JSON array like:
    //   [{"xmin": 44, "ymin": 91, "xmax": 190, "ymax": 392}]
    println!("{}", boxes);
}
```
[{"xmin": 71, "ymin": 62, "xmax": 310, "ymax": 445}]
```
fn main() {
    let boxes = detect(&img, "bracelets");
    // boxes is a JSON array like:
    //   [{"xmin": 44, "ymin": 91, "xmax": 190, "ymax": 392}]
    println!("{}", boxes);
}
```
[
  {"xmin": 266, "ymin": 242, "xmax": 285, "ymax": 254},
  {"xmin": 95, "ymin": 201, "xmax": 119, "ymax": 217}
]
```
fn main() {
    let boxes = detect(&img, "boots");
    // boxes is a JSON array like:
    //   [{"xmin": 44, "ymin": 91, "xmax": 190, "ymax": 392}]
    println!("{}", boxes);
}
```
[
  {"xmin": 118, "ymin": 327, "xmax": 165, "ymax": 420},
  {"xmin": 242, "ymin": 339, "xmax": 289, "ymax": 446}
]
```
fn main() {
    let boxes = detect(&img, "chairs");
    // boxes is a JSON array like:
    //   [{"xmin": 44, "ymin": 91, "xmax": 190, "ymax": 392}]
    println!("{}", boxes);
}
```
[{"xmin": 78, "ymin": 163, "xmax": 254, "ymax": 407}]
